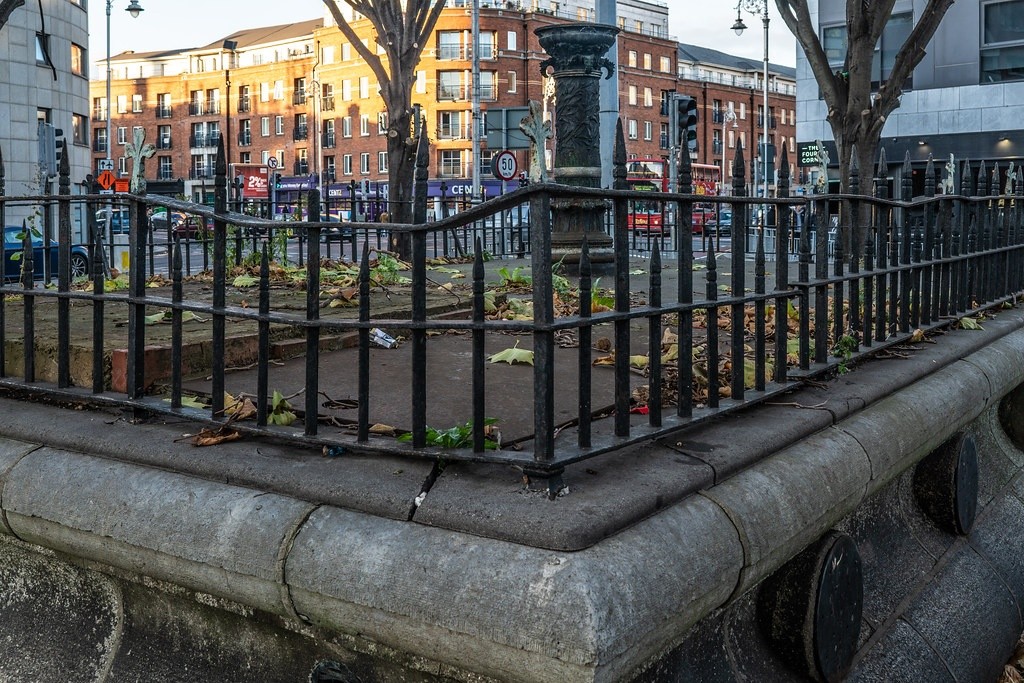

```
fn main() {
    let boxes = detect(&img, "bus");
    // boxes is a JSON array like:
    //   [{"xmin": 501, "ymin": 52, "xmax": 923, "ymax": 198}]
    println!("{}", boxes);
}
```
[{"xmin": 624, "ymin": 159, "xmax": 721, "ymax": 237}]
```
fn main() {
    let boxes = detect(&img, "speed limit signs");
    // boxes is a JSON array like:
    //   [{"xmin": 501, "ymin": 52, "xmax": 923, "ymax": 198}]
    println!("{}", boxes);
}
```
[{"xmin": 496, "ymin": 150, "xmax": 518, "ymax": 181}]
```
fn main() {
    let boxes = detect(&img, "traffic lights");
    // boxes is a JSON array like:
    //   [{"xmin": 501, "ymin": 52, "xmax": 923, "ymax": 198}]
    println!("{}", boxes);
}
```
[{"xmin": 679, "ymin": 99, "xmax": 698, "ymax": 143}]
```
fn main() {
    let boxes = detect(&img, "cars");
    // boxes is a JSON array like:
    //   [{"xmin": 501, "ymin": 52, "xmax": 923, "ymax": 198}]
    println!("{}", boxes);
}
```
[
  {"xmin": 705, "ymin": 212, "xmax": 732, "ymax": 237},
  {"xmin": 297, "ymin": 215, "xmax": 353, "ymax": 243},
  {"xmin": 172, "ymin": 216, "xmax": 214, "ymax": 240},
  {"xmin": 507, "ymin": 204, "xmax": 553, "ymax": 254},
  {"xmin": 3, "ymin": 224, "xmax": 89, "ymax": 284},
  {"xmin": 148, "ymin": 210, "xmax": 188, "ymax": 232},
  {"xmin": 95, "ymin": 207, "xmax": 130, "ymax": 238}
]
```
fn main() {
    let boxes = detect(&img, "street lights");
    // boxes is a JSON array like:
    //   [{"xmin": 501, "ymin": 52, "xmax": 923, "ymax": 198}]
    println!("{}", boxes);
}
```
[
  {"xmin": 105, "ymin": 0, "xmax": 144, "ymax": 269},
  {"xmin": 729, "ymin": 1, "xmax": 770, "ymax": 226}
]
